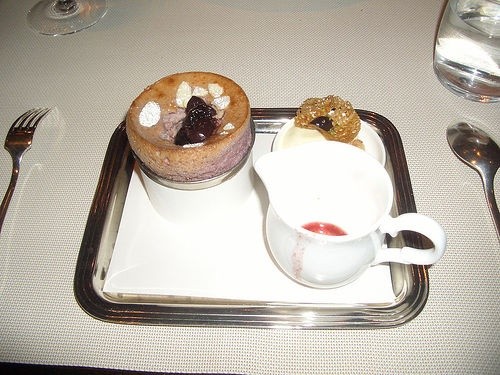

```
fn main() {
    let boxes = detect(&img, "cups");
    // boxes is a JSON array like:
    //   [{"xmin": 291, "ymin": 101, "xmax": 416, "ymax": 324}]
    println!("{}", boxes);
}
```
[
  {"xmin": 434, "ymin": 0, "xmax": 500, "ymax": 105},
  {"xmin": 254, "ymin": 142, "xmax": 446, "ymax": 290}
]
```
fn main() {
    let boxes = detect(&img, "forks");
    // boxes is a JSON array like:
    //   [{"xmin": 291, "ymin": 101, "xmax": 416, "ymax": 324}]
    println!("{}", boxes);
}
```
[{"xmin": 0, "ymin": 106, "xmax": 53, "ymax": 234}]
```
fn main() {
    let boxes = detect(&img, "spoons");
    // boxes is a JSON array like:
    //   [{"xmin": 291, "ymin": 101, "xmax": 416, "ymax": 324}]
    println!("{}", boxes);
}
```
[{"xmin": 446, "ymin": 115, "xmax": 500, "ymax": 243}]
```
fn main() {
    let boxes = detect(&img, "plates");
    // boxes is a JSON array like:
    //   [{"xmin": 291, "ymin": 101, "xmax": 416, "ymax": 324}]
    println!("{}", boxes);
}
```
[
  {"xmin": 133, "ymin": 119, "xmax": 256, "ymax": 190},
  {"xmin": 271, "ymin": 117, "xmax": 386, "ymax": 168}
]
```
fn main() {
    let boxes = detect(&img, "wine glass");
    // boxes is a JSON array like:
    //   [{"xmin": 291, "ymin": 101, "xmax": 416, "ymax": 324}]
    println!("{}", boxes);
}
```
[{"xmin": 26, "ymin": 0, "xmax": 109, "ymax": 35}]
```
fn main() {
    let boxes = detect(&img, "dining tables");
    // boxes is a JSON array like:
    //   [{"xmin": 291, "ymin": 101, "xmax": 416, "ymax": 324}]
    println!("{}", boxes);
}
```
[{"xmin": 0, "ymin": 0, "xmax": 500, "ymax": 375}]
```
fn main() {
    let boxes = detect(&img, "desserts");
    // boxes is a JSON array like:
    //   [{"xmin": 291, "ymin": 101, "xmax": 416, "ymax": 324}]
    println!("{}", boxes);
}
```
[{"xmin": 125, "ymin": 71, "xmax": 252, "ymax": 181}]
